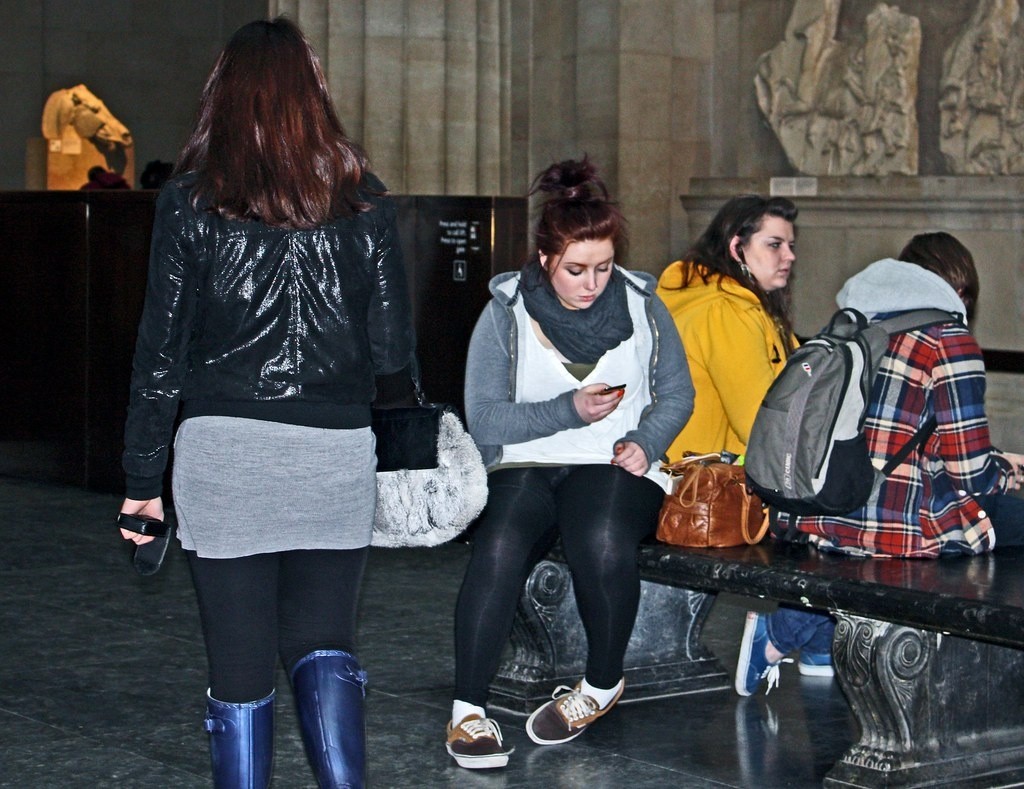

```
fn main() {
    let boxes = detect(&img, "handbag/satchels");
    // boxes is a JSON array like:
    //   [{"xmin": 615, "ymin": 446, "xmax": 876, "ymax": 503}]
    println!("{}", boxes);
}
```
[
  {"xmin": 654, "ymin": 444, "xmax": 770, "ymax": 550},
  {"xmin": 369, "ymin": 392, "xmax": 490, "ymax": 548}
]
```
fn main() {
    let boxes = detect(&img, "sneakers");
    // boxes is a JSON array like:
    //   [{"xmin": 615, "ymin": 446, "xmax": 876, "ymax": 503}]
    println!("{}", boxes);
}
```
[
  {"xmin": 798, "ymin": 651, "xmax": 834, "ymax": 676},
  {"xmin": 734, "ymin": 609, "xmax": 794, "ymax": 696},
  {"xmin": 445, "ymin": 713, "xmax": 510, "ymax": 768},
  {"xmin": 525, "ymin": 678, "xmax": 625, "ymax": 745}
]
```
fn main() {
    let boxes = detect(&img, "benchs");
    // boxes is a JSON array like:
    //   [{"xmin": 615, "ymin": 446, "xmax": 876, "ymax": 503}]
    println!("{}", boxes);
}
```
[{"xmin": 483, "ymin": 529, "xmax": 1023, "ymax": 789}]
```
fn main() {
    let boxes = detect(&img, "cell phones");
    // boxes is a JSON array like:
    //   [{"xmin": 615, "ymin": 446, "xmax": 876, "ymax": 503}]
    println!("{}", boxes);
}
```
[{"xmin": 598, "ymin": 384, "xmax": 627, "ymax": 396}]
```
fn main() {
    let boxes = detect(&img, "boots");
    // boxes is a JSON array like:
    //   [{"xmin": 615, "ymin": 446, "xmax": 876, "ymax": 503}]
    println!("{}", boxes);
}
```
[
  {"xmin": 289, "ymin": 649, "xmax": 369, "ymax": 789},
  {"xmin": 202, "ymin": 687, "xmax": 275, "ymax": 789}
]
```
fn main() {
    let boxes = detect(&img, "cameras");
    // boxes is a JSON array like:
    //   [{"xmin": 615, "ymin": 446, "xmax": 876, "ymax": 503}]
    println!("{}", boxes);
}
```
[{"xmin": 117, "ymin": 514, "xmax": 168, "ymax": 538}]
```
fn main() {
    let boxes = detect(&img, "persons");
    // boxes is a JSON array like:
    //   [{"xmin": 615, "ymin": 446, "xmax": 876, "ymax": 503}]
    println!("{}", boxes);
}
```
[
  {"xmin": 445, "ymin": 160, "xmax": 695, "ymax": 771},
  {"xmin": 79, "ymin": 166, "xmax": 131, "ymax": 190},
  {"xmin": 774, "ymin": 232, "xmax": 1024, "ymax": 560},
  {"xmin": 141, "ymin": 159, "xmax": 175, "ymax": 189},
  {"xmin": 117, "ymin": 19, "xmax": 421, "ymax": 789},
  {"xmin": 656, "ymin": 191, "xmax": 839, "ymax": 695}
]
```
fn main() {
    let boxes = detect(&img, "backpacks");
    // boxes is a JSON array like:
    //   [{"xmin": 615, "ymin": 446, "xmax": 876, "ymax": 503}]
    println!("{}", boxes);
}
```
[{"xmin": 741, "ymin": 308, "xmax": 964, "ymax": 516}]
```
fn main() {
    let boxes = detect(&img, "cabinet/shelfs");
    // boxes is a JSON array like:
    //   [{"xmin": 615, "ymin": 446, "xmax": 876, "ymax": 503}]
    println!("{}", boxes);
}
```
[{"xmin": 2, "ymin": 183, "xmax": 527, "ymax": 501}]
